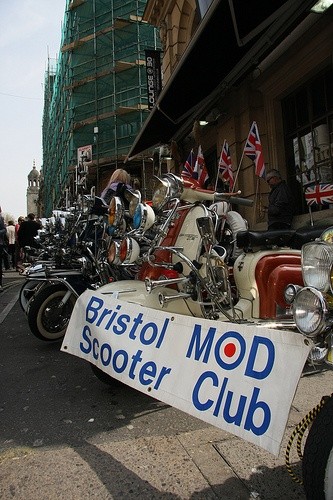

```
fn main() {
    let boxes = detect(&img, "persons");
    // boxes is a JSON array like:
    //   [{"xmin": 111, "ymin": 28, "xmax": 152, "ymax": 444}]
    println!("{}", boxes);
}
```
[
  {"xmin": 6, "ymin": 220, "xmax": 15, "ymax": 269},
  {"xmin": 12, "ymin": 216, "xmax": 25, "ymax": 270},
  {"xmin": 100, "ymin": 168, "xmax": 134, "ymax": 206},
  {"xmin": 204, "ymin": 178, "xmax": 239, "ymax": 212},
  {"xmin": 264, "ymin": 169, "xmax": 292, "ymax": 231},
  {"xmin": 17, "ymin": 213, "xmax": 41, "ymax": 249},
  {"xmin": 0, "ymin": 206, "xmax": 7, "ymax": 288}
]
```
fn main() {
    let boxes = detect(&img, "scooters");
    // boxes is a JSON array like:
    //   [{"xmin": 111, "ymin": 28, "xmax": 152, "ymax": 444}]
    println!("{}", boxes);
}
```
[
  {"xmin": 269, "ymin": 220, "xmax": 333, "ymax": 500},
  {"xmin": 80, "ymin": 170, "xmax": 333, "ymax": 384},
  {"xmin": 17, "ymin": 168, "xmax": 247, "ymax": 344}
]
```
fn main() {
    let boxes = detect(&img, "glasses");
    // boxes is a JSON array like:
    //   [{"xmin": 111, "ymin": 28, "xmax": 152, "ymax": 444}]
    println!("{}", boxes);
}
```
[{"xmin": 266, "ymin": 177, "xmax": 272, "ymax": 182}]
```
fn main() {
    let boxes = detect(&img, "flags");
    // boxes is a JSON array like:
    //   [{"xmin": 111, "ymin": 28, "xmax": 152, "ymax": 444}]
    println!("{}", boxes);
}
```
[{"xmin": 178, "ymin": 119, "xmax": 267, "ymax": 192}]
